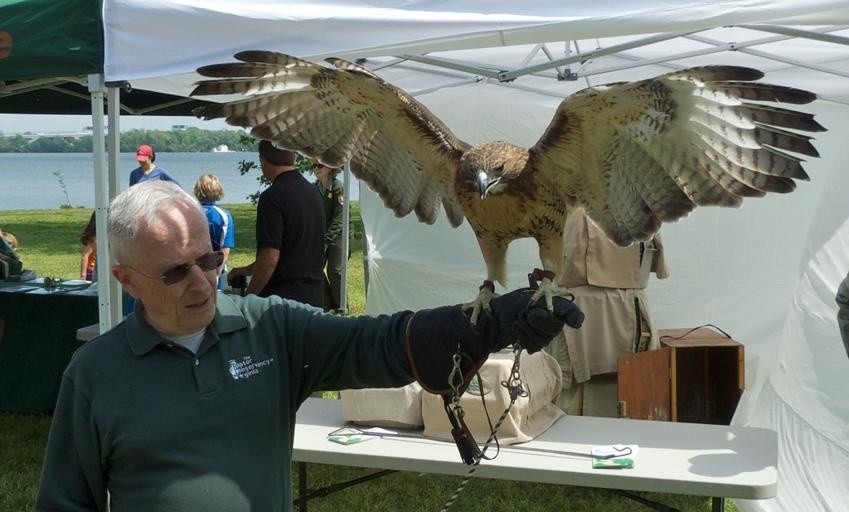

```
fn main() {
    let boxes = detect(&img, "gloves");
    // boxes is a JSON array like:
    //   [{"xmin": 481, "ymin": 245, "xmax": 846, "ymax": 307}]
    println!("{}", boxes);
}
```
[{"xmin": 406, "ymin": 286, "xmax": 585, "ymax": 398}]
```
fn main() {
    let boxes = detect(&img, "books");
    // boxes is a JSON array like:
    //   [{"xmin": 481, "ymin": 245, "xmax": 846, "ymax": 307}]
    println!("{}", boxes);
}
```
[
  {"xmin": 329, "ymin": 426, "xmax": 371, "ymax": 446},
  {"xmin": 593, "ymin": 458, "xmax": 634, "ymax": 470}
]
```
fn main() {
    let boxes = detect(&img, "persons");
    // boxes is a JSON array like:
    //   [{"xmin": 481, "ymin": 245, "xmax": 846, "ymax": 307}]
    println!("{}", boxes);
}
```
[
  {"xmin": 226, "ymin": 139, "xmax": 326, "ymax": 310},
  {"xmin": 129, "ymin": 145, "xmax": 183, "ymax": 188},
  {"xmin": 35, "ymin": 180, "xmax": 585, "ymax": 512},
  {"xmin": 80, "ymin": 210, "xmax": 99, "ymax": 281},
  {"xmin": 309, "ymin": 156, "xmax": 354, "ymax": 313},
  {"xmin": 194, "ymin": 174, "xmax": 241, "ymax": 288}
]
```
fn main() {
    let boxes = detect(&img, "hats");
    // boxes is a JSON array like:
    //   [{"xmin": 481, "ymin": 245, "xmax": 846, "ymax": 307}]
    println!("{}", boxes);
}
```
[{"xmin": 136, "ymin": 145, "xmax": 153, "ymax": 162}]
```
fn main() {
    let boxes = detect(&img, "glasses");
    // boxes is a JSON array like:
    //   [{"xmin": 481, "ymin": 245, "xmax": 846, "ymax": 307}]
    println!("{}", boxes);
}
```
[
  {"xmin": 126, "ymin": 245, "xmax": 224, "ymax": 286},
  {"xmin": 312, "ymin": 163, "xmax": 325, "ymax": 168}
]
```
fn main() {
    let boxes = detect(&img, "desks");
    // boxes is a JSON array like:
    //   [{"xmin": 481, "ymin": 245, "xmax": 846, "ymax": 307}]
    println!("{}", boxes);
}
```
[
  {"xmin": 1, "ymin": 277, "xmax": 99, "ymax": 414},
  {"xmin": 290, "ymin": 396, "xmax": 779, "ymax": 512}
]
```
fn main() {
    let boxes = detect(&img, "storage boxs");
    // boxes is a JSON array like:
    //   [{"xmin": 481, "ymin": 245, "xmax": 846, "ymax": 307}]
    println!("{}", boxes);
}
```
[{"xmin": 617, "ymin": 326, "xmax": 745, "ymax": 424}]
